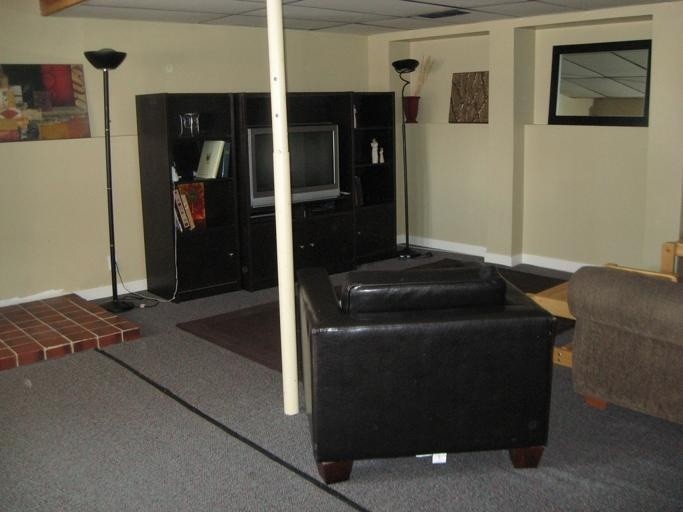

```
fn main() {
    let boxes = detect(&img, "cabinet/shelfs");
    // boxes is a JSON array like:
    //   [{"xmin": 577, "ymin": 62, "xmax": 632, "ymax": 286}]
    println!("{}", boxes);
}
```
[
  {"xmin": 234, "ymin": 94, "xmax": 358, "ymax": 294},
  {"xmin": 349, "ymin": 93, "xmax": 394, "ymax": 262},
  {"xmin": 135, "ymin": 93, "xmax": 241, "ymax": 304}
]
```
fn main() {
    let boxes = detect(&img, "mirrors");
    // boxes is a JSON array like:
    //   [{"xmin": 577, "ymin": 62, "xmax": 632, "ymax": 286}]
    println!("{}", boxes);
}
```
[{"xmin": 547, "ymin": 39, "xmax": 651, "ymax": 127}]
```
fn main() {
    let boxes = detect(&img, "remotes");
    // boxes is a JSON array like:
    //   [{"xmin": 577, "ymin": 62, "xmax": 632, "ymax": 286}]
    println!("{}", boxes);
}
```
[{"xmin": 341, "ymin": 191, "xmax": 351, "ymax": 196}]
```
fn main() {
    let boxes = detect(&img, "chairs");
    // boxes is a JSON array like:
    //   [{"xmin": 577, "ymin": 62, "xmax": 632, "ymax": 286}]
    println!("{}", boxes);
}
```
[
  {"xmin": 565, "ymin": 266, "xmax": 683, "ymax": 425},
  {"xmin": 295, "ymin": 266, "xmax": 558, "ymax": 485}
]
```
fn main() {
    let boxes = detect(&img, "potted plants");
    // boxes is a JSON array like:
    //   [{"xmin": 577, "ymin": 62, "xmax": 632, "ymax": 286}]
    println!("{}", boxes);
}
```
[{"xmin": 404, "ymin": 55, "xmax": 437, "ymax": 123}]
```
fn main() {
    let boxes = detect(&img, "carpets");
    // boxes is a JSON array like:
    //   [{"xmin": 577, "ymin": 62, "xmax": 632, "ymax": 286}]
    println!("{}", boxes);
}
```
[{"xmin": 173, "ymin": 258, "xmax": 568, "ymax": 380}]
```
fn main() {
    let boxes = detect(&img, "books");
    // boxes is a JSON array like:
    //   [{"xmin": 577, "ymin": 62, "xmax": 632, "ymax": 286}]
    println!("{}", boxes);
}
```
[
  {"xmin": 173, "ymin": 188, "xmax": 189, "ymax": 229},
  {"xmin": 352, "ymin": 175, "xmax": 363, "ymax": 209},
  {"xmin": 216, "ymin": 142, "xmax": 231, "ymax": 179},
  {"xmin": 196, "ymin": 141, "xmax": 226, "ymax": 179},
  {"xmin": 174, "ymin": 209, "xmax": 183, "ymax": 231},
  {"xmin": 180, "ymin": 193, "xmax": 195, "ymax": 231}
]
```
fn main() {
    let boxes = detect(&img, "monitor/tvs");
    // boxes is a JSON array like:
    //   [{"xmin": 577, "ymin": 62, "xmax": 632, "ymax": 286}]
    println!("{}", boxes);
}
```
[{"xmin": 247, "ymin": 124, "xmax": 341, "ymax": 209}]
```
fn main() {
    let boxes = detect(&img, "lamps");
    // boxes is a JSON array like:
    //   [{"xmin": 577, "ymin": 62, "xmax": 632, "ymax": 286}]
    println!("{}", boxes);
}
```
[
  {"xmin": 393, "ymin": 59, "xmax": 420, "ymax": 258},
  {"xmin": 84, "ymin": 46, "xmax": 136, "ymax": 313}
]
